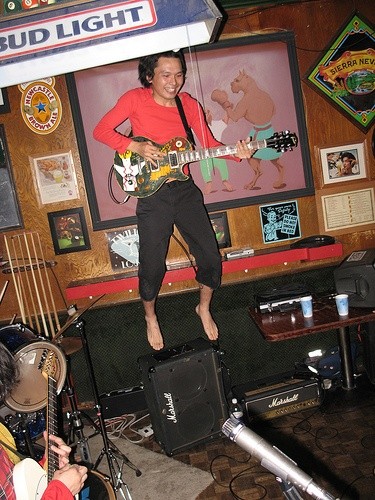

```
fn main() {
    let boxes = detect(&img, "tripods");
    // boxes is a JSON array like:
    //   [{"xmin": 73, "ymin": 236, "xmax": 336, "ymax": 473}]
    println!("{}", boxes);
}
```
[
  {"xmin": 55, "ymin": 345, "xmax": 101, "ymax": 461},
  {"xmin": 76, "ymin": 322, "xmax": 142, "ymax": 491}
]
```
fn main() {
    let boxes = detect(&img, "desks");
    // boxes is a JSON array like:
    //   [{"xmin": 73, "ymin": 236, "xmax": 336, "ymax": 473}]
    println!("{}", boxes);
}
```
[{"xmin": 247, "ymin": 292, "xmax": 375, "ymax": 390}]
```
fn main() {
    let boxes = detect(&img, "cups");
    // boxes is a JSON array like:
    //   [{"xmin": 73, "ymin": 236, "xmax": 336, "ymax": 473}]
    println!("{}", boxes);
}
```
[
  {"xmin": 300, "ymin": 296, "xmax": 313, "ymax": 318},
  {"xmin": 335, "ymin": 294, "xmax": 348, "ymax": 316}
]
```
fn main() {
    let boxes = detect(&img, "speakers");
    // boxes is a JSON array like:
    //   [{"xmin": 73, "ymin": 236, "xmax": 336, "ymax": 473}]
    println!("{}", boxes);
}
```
[
  {"xmin": 334, "ymin": 248, "xmax": 375, "ymax": 309},
  {"xmin": 138, "ymin": 335, "xmax": 231, "ymax": 456}
]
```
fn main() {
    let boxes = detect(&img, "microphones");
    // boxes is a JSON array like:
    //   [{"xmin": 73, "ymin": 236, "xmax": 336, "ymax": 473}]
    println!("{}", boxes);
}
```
[{"xmin": 218, "ymin": 415, "xmax": 340, "ymax": 500}]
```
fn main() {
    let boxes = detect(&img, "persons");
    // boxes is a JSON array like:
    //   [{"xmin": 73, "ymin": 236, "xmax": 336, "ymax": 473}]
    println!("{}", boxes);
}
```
[
  {"xmin": 0, "ymin": 345, "xmax": 87, "ymax": 500},
  {"xmin": 326, "ymin": 152, "xmax": 359, "ymax": 176},
  {"xmin": 91, "ymin": 49, "xmax": 256, "ymax": 350}
]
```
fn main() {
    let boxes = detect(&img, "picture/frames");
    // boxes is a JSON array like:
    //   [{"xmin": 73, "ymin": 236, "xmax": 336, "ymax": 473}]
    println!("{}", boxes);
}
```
[
  {"xmin": 30, "ymin": 148, "xmax": 80, "ymax": 209},
  {"xmin": 301, "ymin": 10, "xmax": 375, "ymax": 135},
  {"xmin": 0, "ymin": 123, "xmax": 25, "ymax": 230},
  {"xmin": 313, "ymin": 139, "xmax": 371, "ymax": 191},
  {"xmin": 47, "ymin": 206, "xmax": 91, "ymax": 256},
  {"xmin": 61, "ymin": 32, "xmax": 315, "ymax": 230},
  {"xmin": 190, "ymin": 211, "xmax": 232, "ymax": 252}
]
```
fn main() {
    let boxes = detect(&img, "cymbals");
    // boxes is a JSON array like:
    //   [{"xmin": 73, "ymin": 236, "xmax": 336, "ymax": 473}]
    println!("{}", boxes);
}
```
[{"xmin": 50, "ymin": 291, "xmax": 106, "ymax": 342}]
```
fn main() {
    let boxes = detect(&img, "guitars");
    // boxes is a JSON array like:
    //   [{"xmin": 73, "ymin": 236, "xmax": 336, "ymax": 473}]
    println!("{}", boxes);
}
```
[
  {"xmin": 13, "ymin": 348, "xmax": 116, "ymax": 500},
  {"xmin": 113, "ymin": 131, "xmax": 297, "ymax": 197}
]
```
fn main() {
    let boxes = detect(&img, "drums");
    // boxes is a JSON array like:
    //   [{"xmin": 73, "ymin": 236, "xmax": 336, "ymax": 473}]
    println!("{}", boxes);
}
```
[{"xmin": 0, "ymin": 324, "xmax": 66, "ymax": 412}]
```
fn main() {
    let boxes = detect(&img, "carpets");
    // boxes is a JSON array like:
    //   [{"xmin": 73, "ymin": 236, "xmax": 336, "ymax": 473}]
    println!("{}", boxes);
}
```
[{"xmin": 75, "ymin": 426, "xmax": 216, "ymax": 500}]
